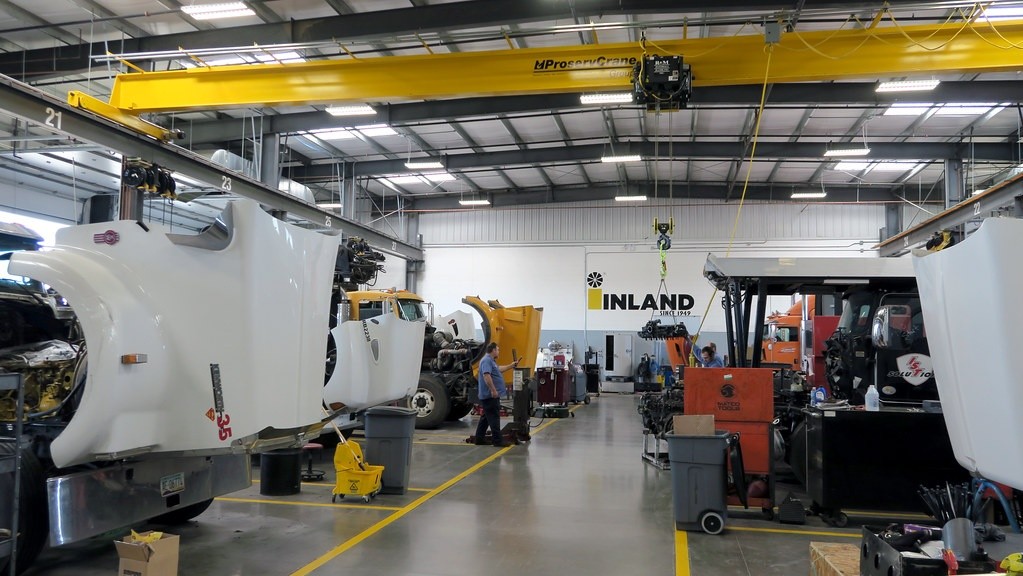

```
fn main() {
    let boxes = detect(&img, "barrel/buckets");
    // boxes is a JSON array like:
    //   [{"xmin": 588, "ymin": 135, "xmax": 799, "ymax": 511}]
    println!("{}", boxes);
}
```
[{"xmin": 260, "ymin": 451, "xmax": 301, "ymax": 496}]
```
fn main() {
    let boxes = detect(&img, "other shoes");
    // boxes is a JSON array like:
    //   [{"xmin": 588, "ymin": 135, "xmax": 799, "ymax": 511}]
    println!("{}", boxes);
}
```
[
  {"xmin": 493, "ymin": 439, "xmax": 510, "ymax": 447},
  {"xmin": 476, "ymin": 438, "xmax": 492, "ymax": 445}
]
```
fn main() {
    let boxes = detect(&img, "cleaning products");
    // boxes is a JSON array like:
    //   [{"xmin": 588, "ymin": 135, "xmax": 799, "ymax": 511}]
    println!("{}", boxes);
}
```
[{"xmin": 814, "ymin": 385, "xmax": 826, "ymax": 406}]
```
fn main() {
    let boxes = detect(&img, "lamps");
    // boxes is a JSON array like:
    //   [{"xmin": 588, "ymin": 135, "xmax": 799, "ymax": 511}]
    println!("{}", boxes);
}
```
[
  {"xmin": 822, "ymin": 127, "xmax": 871, "ymax": 156},
  {"xmin": 458, "ymin": 179, "xmax": 491, "ymax": 205},
  {"xmin": 601, "ymin": 137, "xmax": 642, "ymax": 162},
  {"xmin": 614, "ymin": 179, "xmax": 647, "ymax": 201},
  {"xmin": 791, "ymin": 173, "xmax": 826, "ymax": 198},
  {"xmin": 404, "ymin": 141, "xmax": 444, "ymax": 169}
]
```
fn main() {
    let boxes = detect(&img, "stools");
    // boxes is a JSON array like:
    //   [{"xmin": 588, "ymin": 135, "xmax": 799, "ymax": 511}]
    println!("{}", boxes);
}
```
[{"xmin": 301, "ymin": 443, "xmax": 326, "ymax": 481}]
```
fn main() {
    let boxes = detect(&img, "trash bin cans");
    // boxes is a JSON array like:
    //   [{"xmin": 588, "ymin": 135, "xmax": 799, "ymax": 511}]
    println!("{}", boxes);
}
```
[
  {"xmin": 537, "ymin": 368, "xmax": 569, "ymax": 405},
  {"xmin": 666, "ymin": 429, "xmax": 749, "ymax": 536},
  {"xmin": 570, "ymin": 366, "xmax": 590, "ymax": 405},
  {"xmin": 363, "ymin": 405, "xmax": 419, "ymax": 496}
]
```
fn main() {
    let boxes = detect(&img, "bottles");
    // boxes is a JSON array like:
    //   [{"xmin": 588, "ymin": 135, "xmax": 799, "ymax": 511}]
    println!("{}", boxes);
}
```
[
  {"xmin": 865, "ymin": 385, "xmax": 880, "ymax": 411},
  {"xmin": 811, "ymin": 387, "xmax": 816, "ymax": 407},
  {"xmin": 814, "ymin": 385, "xmax": 827, "ymax": 404}
]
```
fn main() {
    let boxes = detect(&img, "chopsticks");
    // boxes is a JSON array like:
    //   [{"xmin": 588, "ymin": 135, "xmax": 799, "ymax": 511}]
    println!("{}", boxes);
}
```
[{"xmin": 917, "ymin": 482, "xmax": 991, "ymax": 527}]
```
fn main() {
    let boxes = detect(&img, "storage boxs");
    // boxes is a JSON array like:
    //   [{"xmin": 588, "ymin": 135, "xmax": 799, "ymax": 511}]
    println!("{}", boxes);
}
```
[
  {"xmin": 672, "ymin": 415, "xmax": 714, "ymax": 435},
  {"xmin": 112, "ymin": 531, "xmax": 179, "ymax": 576}
]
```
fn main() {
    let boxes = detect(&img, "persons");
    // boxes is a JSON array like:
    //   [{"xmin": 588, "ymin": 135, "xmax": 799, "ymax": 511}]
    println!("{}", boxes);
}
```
[
  {"xmin": 689, "ymin": 334, "xmax": 724, "ymax": 368},
  {"xmin": 474, "ymin": 342, "xmax": 517, "ymax": 447}
]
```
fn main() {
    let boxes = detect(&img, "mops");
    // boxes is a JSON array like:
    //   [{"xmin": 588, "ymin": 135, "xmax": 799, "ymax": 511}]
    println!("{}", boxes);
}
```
[{"xmin": 322, "ymin": 406, "xmax": 366, "ymax": 471}]
